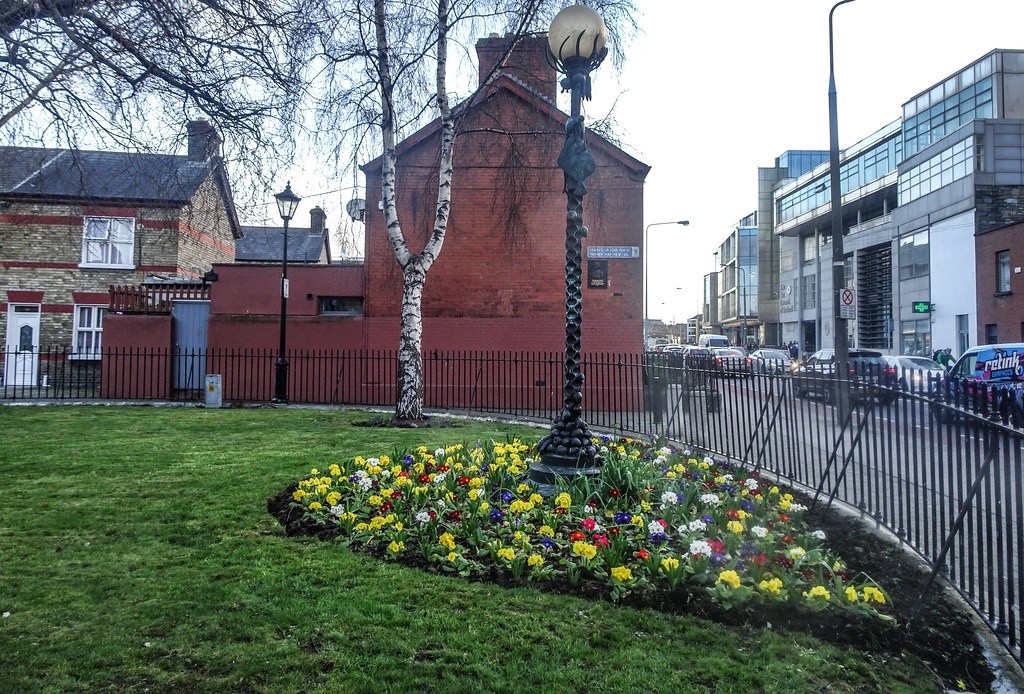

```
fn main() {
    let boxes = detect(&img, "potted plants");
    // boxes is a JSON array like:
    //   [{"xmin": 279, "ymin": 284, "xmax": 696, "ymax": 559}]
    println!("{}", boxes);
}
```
[{"xmin": 644, "ymin": 358, "xmax": 722, "ymax": 414}]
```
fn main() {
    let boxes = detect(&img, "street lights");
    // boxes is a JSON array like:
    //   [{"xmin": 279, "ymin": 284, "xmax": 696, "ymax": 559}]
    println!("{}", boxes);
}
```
[
  {"xmin": 269, "ymin": 180, "xmax": 302, "ymax": 406},
  {"xmin": 645, "ymin": 220, "xmax": 691, "ymax": 353},
  {"xmin": 522, "ymin": 3, "xmax": 607, "ymax": 503}
]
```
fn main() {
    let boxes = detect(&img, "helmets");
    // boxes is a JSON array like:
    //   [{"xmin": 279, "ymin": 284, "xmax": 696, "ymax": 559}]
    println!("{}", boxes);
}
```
[{"xmin": 944, "ymin": 346, "xmax": 952, "ymax": 351}]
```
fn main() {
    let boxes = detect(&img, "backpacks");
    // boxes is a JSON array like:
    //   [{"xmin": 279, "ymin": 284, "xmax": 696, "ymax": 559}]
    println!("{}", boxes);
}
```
[{"xmin": 933, "ymin": 349, "xmax": 943, "ymax": 361}]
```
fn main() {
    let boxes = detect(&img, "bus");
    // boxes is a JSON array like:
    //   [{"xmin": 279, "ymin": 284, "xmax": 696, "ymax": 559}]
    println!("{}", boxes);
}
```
[{"xmin": 698, "ymin": 333, "xmax": 730, "ymax": 348}]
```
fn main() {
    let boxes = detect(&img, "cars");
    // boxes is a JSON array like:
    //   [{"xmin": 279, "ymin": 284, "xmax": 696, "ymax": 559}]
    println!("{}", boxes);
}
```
[
  {"xmin": 885, "ymin": 354, "xmax": 950, "ymax": 401},
  {"xmin": 790, "ymin": 346, "xmax": 895, "ymax": 406},
  {"xmin": 926, "ymin": 340, "xmax": 1024, "ymax": 441},
  {"xmin": 749, "ymin": 349, "xmax": 796, "ymax": 379},
  {"xmin": 646, "ymin": 343, "xmax": 751, "ymax": 379}
]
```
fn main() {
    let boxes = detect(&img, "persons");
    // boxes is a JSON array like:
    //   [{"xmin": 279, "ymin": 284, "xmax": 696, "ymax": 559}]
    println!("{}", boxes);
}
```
[
  {"xmin": 741, "ymin": 341, "xmax": 758, "ymax": 352},
  {"xmin": 936, "ymin": 348, "xmax": 956, "ymax": 374},
  {"xmin": 781, "ymin": 340, "xmax": 798, "ymax": 358}
]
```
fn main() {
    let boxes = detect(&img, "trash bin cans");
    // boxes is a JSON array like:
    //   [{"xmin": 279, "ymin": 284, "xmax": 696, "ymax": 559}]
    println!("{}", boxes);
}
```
[
  {"xmin": 646, "ymin": 353, "xmax": 669, "ymax": 390},
  {"xmin": 205, "ymin": 374, "xmax": 223, "ymax": 409}
]
